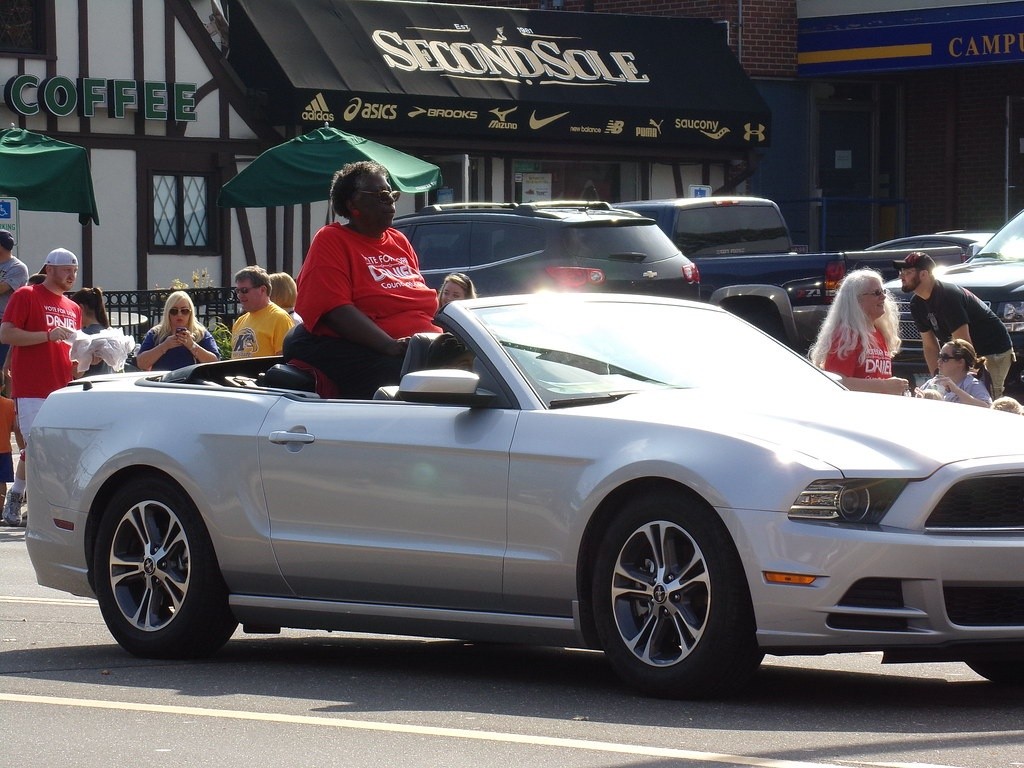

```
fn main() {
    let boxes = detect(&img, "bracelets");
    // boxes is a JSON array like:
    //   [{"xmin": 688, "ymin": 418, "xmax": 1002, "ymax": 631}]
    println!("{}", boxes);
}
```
[
  {"xmin": 159, "ymin": 343, "xmax": 166, "ymax": 355},
  {"xmin": 189, "ymin": 341, "xmax": 196, "ymax": 350},
  {"xmin": 965, "ymin": 395, "xmax": 974, "ymax": 403},
  {"xmin": 195, "ymin": 345, "xmax": 200, "ymax": 358},
  {"xmin": 47, "ymin": 329, "xmax": 52, "ymax": 341}
]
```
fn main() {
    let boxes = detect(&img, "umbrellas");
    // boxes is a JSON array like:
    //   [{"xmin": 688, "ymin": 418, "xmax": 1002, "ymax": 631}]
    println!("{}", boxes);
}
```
[
  {"xmin": 0, "ymin": 127, "xmax": 101, "ymax": 227},
  {"xmin": 219, "ymin": 123, "xmax": 444, "ymax": 226}
]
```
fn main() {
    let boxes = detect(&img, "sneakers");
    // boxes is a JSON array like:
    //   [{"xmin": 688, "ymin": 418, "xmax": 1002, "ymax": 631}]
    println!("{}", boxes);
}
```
[{"xmin": 2, "ymin": 487, "xmax": 27, "ymax": 528}]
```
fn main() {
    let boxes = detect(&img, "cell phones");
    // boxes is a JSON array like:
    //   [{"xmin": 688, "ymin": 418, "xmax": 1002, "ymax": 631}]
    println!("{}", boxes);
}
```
[{"xmin": 175, "ymin": 327, "xmax": 187, "ymax": 336}]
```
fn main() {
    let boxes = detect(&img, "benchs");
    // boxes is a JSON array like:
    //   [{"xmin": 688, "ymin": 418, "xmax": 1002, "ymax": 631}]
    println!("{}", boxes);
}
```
[{"xmin": 262, "ymin": 367, "xmax": 327, "ymax": 398}]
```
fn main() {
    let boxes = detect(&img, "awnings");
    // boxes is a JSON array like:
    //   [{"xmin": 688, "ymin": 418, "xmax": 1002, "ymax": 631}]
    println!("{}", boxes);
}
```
[{"xmin": 219, "ymin": 0, "xmax": 772, "ymax": 150}]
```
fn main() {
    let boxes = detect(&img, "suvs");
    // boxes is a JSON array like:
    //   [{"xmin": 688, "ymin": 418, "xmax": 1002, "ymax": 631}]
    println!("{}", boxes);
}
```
[
  {"xmin": 390, "ymin": 200, "xmax": 702, "ymax": 311},
  {"xmin": 883, "ymin": 207, "xmax": 1024, "ymax": 401}
]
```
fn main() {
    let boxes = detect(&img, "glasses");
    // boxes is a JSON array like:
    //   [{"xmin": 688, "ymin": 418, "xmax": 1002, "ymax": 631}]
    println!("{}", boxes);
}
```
[
  {"xmin": 859, "ymin": 289, "xmax": 887, "ymax": 296},
  {"xmin": 938, "ymin": 352, "xmax": 964, "ymax": 362},
  {"xmin": 169, "ymin": 309, "xmax": 192, "ymax": 316},
  {"xmin": 357, "ymin": 190, "xmax": 401, "ymax": 203},
  {"xmin": 235, "ymin": 286, "xmax": 257, "ymax": 294}
]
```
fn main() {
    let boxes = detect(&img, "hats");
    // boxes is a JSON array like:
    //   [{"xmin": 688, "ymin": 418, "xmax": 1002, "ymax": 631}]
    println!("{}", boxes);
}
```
[
  {"xmin": 0, "ymin": 229, "xmax": 14, "ymax": 250},
  {"xmin": 892, "ymin": 252, "xmax": 936, "ymax": 273},
  {"xmin": 38, "ymin": 248, "xmax": 79, "ymax": 274}
]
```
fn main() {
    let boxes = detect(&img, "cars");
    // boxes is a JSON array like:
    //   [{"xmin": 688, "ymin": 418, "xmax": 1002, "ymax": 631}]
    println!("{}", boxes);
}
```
[
  {"xmin": 28, "ymin": 293, "xmax": 1024, "ymax": 702},
  {"xmin": 864, "ymin": 230, "xmax": 996, "ymax": 262}
]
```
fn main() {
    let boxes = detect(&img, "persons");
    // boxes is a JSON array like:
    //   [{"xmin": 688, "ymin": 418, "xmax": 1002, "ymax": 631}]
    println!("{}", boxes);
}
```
[
  {"xmin": 437, "ymin": 273, "xmax": 477, "ymax": 307},
  {"xmin": 808, "ymin": 251, "xmax": 1024, "ymax": 417},
  {"xmin": 0, "ymin": 230, "xmax": 303, "ymax": 526},
  {"xmin": 294, "ymin": 160, "xmax": 443, "ymax": 401}
]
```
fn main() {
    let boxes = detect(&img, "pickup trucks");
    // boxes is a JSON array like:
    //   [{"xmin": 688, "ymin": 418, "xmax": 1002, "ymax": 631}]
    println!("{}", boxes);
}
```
[{"xmin": 610, "ymin": 195, "xmax": 964, "ymax": 360}]
